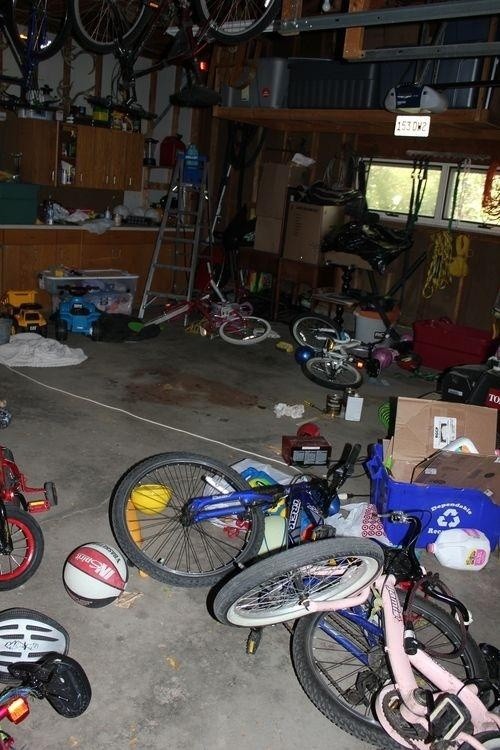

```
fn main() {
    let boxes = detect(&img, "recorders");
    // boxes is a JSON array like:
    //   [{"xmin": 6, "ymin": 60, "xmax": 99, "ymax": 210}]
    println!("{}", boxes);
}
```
[{"xmin": 384, "ymin": 81, "xmax": 448, "ymax": 113}]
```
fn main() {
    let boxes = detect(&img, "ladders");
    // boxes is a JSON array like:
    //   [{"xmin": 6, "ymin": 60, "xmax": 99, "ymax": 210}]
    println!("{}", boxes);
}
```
[{"xmin": 138, "ymin": 151, "xmax": 209, "ymax": 327}]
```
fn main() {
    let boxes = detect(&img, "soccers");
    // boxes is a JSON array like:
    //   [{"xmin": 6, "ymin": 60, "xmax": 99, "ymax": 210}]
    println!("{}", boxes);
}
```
[{"xmin": 295, "ymin": 346, "xmax": 315, "ymax": 364}]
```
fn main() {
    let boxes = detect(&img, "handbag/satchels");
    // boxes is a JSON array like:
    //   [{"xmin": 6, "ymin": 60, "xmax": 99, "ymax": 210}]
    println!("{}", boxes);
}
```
[{"xmin": 308, "ymin": 180, "xmax": 357, "ymax": 205}]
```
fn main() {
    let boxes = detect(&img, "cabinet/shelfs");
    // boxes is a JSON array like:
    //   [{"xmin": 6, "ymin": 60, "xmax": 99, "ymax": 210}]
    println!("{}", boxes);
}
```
[
  {"xmin": 97, "ymin": 128, "xmax": 123, "ymax": 195},
  {"xmin": 153, "ymin": 229, "xmax": 200, "ymax": 306},
  {"xmin": 41, "ymin": 120, "xmax": 97, "ymax": 190},
  {"xmin": 0, "ymin": 117, "xmax": 40, "ymax": 190},
  {"xmin": 0, "ymin": 228, "xmax": 82, "ymax": 305},
  {"xmin": 209, "ymin": 0, "xmax": 500, "ymax": 152},
  {"xmin": 122, "ymin": 128, "xmax": 146, "ymax": 190},
  {"xmin": 83, "ymin": 227, "xmax": 152, "ymax": 315}
]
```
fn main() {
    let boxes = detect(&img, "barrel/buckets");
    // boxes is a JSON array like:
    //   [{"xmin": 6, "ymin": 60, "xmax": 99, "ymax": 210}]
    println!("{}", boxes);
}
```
[{"xmin": 352, "ymin": 308, "xmax": 399, "ymax": 346}]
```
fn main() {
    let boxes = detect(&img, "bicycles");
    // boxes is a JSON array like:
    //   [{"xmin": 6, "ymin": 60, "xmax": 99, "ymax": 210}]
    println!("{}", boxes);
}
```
[
  {"xmin": 213, "ymin": 510, "xmax": 500, "ymax": 750},
  {"xmin": 142, "ymin": 263, "xmax": 272, "ymax": 346},
  {"xmin": 110, "ymin": 444, "xmax": 500, "ymax": 750},
  {"xmin": 0, "ymin": 405, "xmax": 59, "ymax": 594},
  {"xmin": 295, "ymin": 311, "xmax": 394, "ymax": 390},
  {"xmin": 0, "ymin": 0, "xmax": 286, "ymax": 119}
]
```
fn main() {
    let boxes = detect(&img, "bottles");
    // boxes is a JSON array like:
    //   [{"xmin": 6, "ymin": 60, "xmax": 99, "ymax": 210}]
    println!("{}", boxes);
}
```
[
  {"xmin": 62, "ymin": 165, "xmax": 72, "ymax": 186},
  {"xmin": 47, "ymin": 205, "xmax": 55, "ymax": 226},
  {"xmin": 105, "ymin": 208, "xmax": 123, "ymax": 227}
]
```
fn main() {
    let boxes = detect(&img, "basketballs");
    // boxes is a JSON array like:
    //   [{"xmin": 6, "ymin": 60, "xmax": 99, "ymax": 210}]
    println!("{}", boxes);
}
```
[{"xmin": 63, "ymin": 544, "xmax": 128, "ymax": 609}]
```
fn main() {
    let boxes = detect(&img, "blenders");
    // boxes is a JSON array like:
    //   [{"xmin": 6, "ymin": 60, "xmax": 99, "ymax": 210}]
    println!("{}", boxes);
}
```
[{"xmin": 144, "ymin": 137, "xmax": 159, "ymax": 167}]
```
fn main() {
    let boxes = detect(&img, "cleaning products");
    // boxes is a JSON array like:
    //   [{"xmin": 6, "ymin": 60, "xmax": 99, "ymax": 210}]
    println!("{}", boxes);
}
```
[{"xmin": 428, "ymin": 527, "xmax": 491, "ymax": 573}]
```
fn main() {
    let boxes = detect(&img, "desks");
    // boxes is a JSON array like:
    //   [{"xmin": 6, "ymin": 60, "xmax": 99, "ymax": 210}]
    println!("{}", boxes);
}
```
[
  {"xmin": 271, "ymin": 254, "xmax": 343, "ymax": 329},
  {"xmin": 235, "ymin": 244, "xmax": 281, "ymax": 321}
]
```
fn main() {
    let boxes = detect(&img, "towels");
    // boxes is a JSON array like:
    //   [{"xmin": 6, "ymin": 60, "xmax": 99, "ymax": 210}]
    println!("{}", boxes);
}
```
[{"xmin": 0, "ymin": 331, "xmax": 87, "ymax": 367}]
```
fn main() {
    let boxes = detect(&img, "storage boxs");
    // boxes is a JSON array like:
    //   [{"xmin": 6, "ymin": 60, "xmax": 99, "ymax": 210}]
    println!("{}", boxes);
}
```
[
  {"xmin": 40, "ymin": 267, "xmax": 139, "ymax": 313},
  {"xmin": 410, "ymin": 318, "xmax": 493, "ymax": 372},
  {"xmin": 1, "ymin": 183, "xmax": 39, "ymax": 225},
  {"xmin": 215, "ymin": 57, "xmax": 287, "ymax": 107},
  {"xmin": 395, "ymin": 55, "xmax": 484, "ymax": 107},
  {"xmin": 254, "ymin": 160, "xmax": 301, "ymax": 217},
  {"xmin": 384, "ymin": 397, "xmax": 499, "ymax": 487},
  {"xmin": 249, "ymin": 214, "xmax": 284, "ymax": 253},
  {"xmin": 285, "ymin": 206, "xmax": 346, "ymax": 266},
  {"xmin": 366, "ymin": 446, "xmax": 499, "ymax": 553},
  {"xmin": 288, "ymin": 58, "xmax": 381, "ymax": 109}
]
